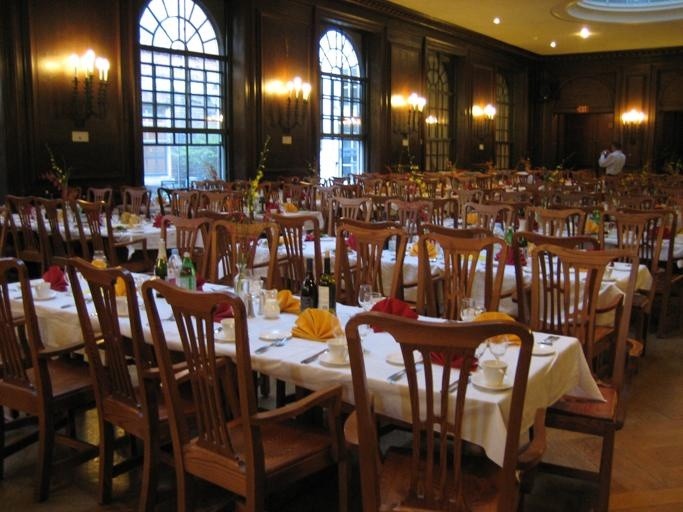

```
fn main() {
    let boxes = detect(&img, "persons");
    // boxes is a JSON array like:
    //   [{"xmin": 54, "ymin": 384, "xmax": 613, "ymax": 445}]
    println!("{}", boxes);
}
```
[{"xmin": 598, "ymin": 140, "xmax": 626, "ymax": 178}]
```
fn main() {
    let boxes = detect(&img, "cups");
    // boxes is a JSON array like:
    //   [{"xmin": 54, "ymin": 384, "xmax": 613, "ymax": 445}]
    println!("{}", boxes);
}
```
[
  {"xmin": 263, "ymin": 300, "xmax": 280, "ymax": 318},
  {"xmin": 33, "ymin": 281, "xmax": 50, "ymax": 297},
  {"xmin": 115, "ymin": 295, "xmax": 129, "ymax": 316},
  {"xmin": 358, "ymin": 284, "xmax": 380, "ymax": 312},
  {"xmin": 459, "ymin": 296, "xmax": 484, "ymax": 322},
  {"xmin": 480, "ymin": 359, "xmax": 509, "ymax": 387},
  {"xmin": 327, "ymin": 337, "xmax": 349, "ymax": 362},
  {"xmin": 221, "ymin": 317, "xmax": 237, "ymax": 339}
]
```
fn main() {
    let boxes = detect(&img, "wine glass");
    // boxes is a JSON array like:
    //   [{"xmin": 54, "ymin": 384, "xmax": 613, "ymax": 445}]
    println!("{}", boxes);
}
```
[{"xmin": 471, "ymin": 332, "xmax": 508, "ymax": 359}]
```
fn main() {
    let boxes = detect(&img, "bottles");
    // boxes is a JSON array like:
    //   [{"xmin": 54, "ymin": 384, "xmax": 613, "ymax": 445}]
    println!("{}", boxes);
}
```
[
  {"xmin": 299, "ymin": 246, "xmax": 336, "ymax": 312},
  {"xmin": 242, "ymin": 291, "xmax": 255, "ymax": 320},
  {"xmin": 257, "ymin": 291, "xmax": 267, "ymax": 316},
  {"xmin": 92, "ymin": 250, "xmax": 106, "ymax": 262},
  {"xmin": 154, "ymin": 236, "xmax": 196, "ymax": 298}
]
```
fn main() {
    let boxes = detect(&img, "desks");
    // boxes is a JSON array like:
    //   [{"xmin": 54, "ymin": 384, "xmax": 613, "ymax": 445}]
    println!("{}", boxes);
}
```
[{"xmin": 3, "ymin": 268, "xmax": 613, "ymax": 470}]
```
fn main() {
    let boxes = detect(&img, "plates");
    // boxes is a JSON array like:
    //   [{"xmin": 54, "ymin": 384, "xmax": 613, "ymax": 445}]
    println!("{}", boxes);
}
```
[
  {"xmin": 211, "ymin": 332, "xmax": 240, "ymax": 342},
  {"xmin": 260, "ymin": 328, "xmax": 291, "ymax": 340},
  {"xmin": 470, "ymin": 373, "xmax": 518, "ymax": 392},
  {"xmin": 383, "ymin": 349, "xmax": 424, "ymax": 366},
  {"xmin": 32, "ymin": 289, "xmax": 55, "ymax": 301},
  {"xmin": 263, "ymin": 229, "xmax": 642, "ymax": 286},
  {"xmin": 525, "ymin": 344, "xmax": 554, "ymax": 356},
  {"xmin": 1, "ymin": 206, "xmax": 170, "ymax": 236},
  {"xmin": 317, "ymin": 352, "xmax": 357, "ymax": 366}
]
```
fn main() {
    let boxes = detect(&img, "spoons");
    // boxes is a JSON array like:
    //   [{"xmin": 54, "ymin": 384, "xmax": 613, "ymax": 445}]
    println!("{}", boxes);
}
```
[
  {"xmin": 302, "ymin": 349, "xmax": 329, "ymax": 364},
  {"xmin": 385, "ymin": 366, "xmax": 422, "ymax": 385},
  {"xmin": 540, "ymin": 334, "xmax": 558, "ymax": 347},
  {"xmin": 256, "ymin": 336, "xmax": 289, "ymax": 355}
]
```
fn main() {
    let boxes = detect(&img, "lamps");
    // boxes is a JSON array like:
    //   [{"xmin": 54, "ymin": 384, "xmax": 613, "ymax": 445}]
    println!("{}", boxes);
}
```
[
  {"xmin": 273, "ymin": 77, "xmax": 312, "ymax": 145},
  {"xmin": 471, "ymin": 104, "xmax": 496, "ymax": 151},
  {"xmin": 69, "ymin": 51, "xmax": 110, "ymax": 142},
  {"xmin": 621, "ymin": 109, "xmax": 646, "ymax": 145},
  {"xmin": 402, "ymin": 92, "xmax": 437, "ymax": 146}
]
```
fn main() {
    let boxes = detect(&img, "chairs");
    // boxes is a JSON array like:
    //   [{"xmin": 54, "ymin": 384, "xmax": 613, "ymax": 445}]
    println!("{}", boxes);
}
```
[
  {"xmin": 0, "ymin": 167, "xmax": 683, "ymax": 386},
  {"xmin": 0, "ymin": 255, "xmax": 124, "ymax": 512},
  {"xmin": 141, "ymin": 276, "xmax": 351, "ymax": 512},
  {"xmin": 65, "ymin": 255, "xmax": 199, "ymax": 512},
  {"xmin": 334, "ymin": 311, "xmax": 535, "ymax": 512},
  {"xmin": 517, "ymin": 244, "xmax": 644, "ymax": 512}
]
